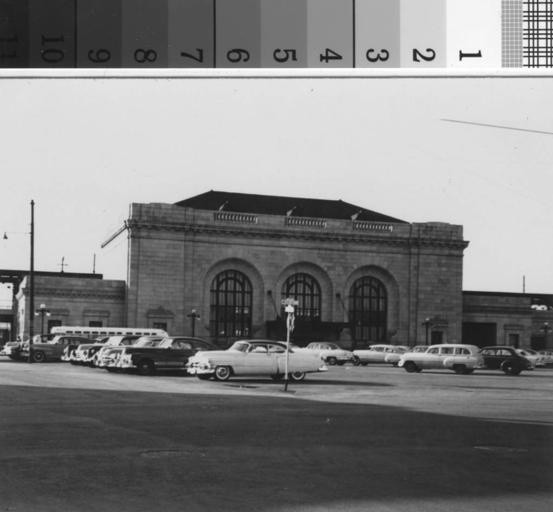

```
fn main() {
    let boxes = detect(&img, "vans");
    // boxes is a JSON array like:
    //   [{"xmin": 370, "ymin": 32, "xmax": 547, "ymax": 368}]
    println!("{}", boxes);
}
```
[{"xmin": 350, "ymin": 343, "xmax": 405, "ymax": 367}]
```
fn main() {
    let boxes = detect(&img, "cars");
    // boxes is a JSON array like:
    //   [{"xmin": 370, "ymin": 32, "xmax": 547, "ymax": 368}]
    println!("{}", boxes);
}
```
[
  {"xmin": 383, "ymin": 345, "xmax": 428, "ymax": 366},
  {"xmin": 1, "ymin": 336, "xmax": 223, "ymax": 375},
  {"xmin": 186, "ymin": 338, "xmax": 328, "ymax": 381},
  {"xmin": 478, "ymin": 344, "xmax": 552, "ymax": 375},
  {"xmin": 305, "ymin": 341, "xmax": 354, "ymax": 365}
]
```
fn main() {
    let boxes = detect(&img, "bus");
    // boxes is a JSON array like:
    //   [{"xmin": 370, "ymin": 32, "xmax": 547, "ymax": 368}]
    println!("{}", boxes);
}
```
[{"xmin": 50, "ymin": 325, "xmax": 168, "ymax": 338}]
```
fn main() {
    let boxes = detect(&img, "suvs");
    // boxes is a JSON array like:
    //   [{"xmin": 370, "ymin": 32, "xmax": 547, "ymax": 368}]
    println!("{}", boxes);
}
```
[{"xmin": 396, "ymin": 343, "xmax": 485, "ymax": 375}]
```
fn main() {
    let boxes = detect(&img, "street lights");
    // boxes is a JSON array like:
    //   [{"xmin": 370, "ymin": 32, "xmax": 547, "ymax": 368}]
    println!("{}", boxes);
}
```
[
  {"xmin": 35, "ymin": 302, "xmax": 52, "ymax": 341},
  {"xmin": 185, "ymin": 308, "xmax": 202, "ymax": 337}
]
